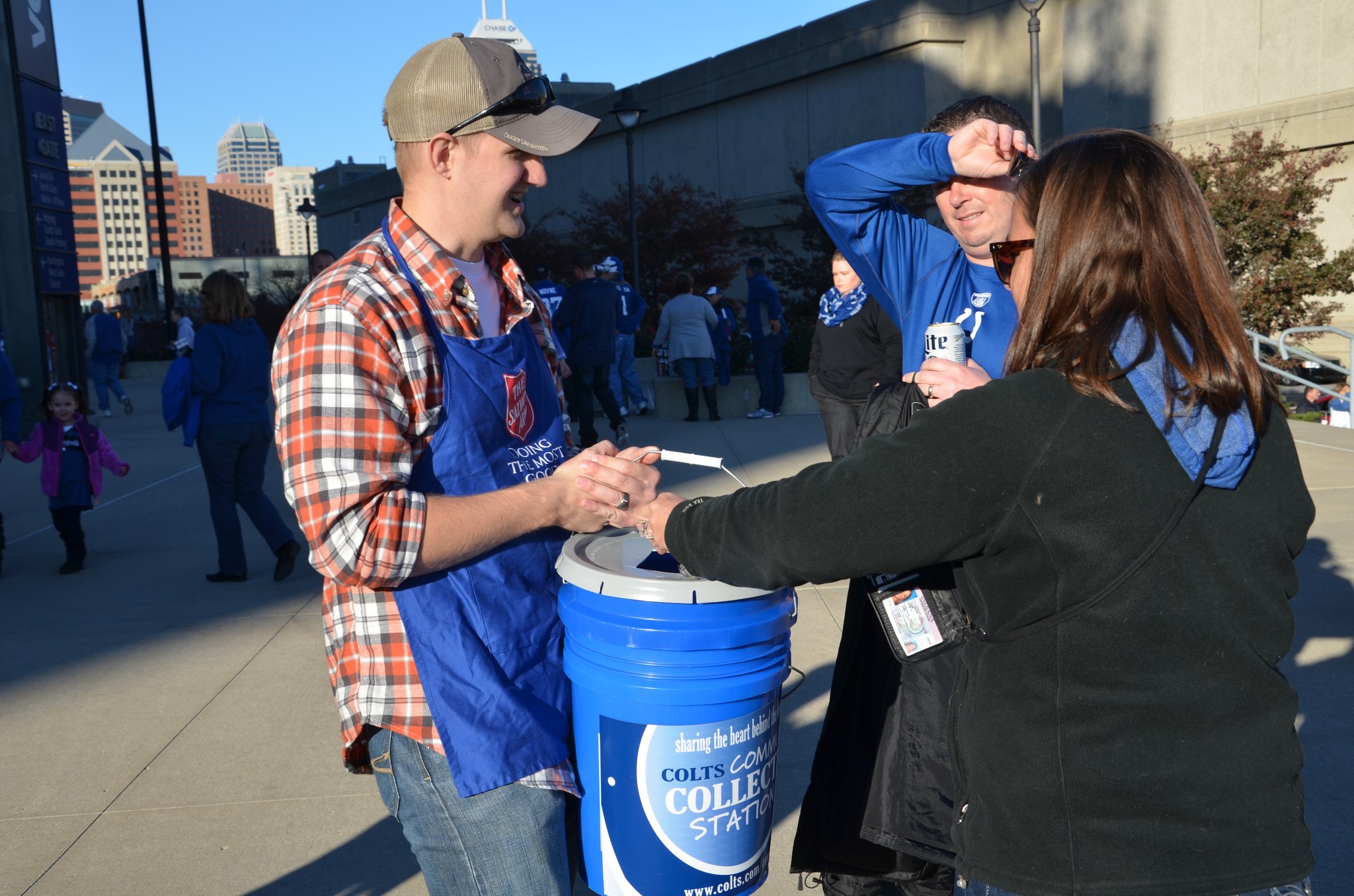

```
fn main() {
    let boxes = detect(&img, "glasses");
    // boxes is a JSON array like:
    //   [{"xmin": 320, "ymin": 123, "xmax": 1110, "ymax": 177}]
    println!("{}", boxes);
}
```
[
  {"xmin": 989, "ymin": 239, "xmax": 1036, "ymax": 286},
  {"xmin": 445, "ymin": 74, "xmax": 557, "ymax": 136}
]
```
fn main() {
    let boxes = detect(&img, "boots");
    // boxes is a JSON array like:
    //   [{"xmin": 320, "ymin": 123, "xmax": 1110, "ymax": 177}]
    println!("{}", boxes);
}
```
[
  {"xmin": 682, "ymin": 387, "xmax": 699, "ymax": 422},
  {"xmin": 702, "ymin": 384, "xmax": 721, "ymax": 422}
]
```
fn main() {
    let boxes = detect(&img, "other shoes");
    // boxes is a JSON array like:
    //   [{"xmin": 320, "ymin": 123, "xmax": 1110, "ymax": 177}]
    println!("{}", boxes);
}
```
[{"xmin": 568, "ymin": 413, "xmax": 578, "ymax": 423}]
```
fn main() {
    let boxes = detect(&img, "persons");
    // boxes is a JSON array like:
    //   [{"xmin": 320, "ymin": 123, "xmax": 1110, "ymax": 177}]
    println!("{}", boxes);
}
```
[
  {"xmin": 81, "ymin": 298, "xmax": 136, "ymax": 418},
  {"xmin": 530, "ymin": 249, "xmax": 650, "ymax": 449},
  {"xmin": 0, "ymin": 334, "xmax": 27, "ymax": 574},
  {"xmin": 169, "ymin": 305, "xmax": 197, "ymax": 358},
  {"xmin": 652, "ymin": 272, "xmax": 723, "ymax": 421},
  {"xmin": 1296, "ymin": 385, "xmax": 1322, "ymax": 422},
  {"xmin": 608, "ymin": 127, "xmax": 1318, "ymax": 896},
  {"xmin": 159, "ymin": 268, "xmax": 301, "ymax": 582},
  {"xmin": 239, "ymin": 28, "xmax": 661, "ymax": 896},
  {"xmin": 704, "ymin": 287, "xmax": 736, "ymax": 387},
  {"xmin": 8, "ymin": 377, "xmax": 132, "ymax": 575},
  {"xmin": 744, "ymin": 255, "xmax": 786, "ymax": 418},
  {"xmin": 787, "ymin": 94, "xmax": 1042, "ymax": 896},
  {"xmin": 807, "ymin": 251, "xmax": 904, "ymax": 463},
  {"xmin": 292, "ymin": 249, "xmax": 337, "ymax": 309},
  {"xmin": 1327, "ymin": 382, "xmax": 1351, "ymax": 427}
]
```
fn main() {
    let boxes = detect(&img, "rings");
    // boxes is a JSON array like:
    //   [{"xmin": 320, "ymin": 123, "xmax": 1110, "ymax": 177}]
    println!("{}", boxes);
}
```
[
  {"xmin": 638, "ymin": 522, "xmax": 653, "ymax": 540},
  {"xmin": 613, "ymin": 491, "xmax": 630, "ymax": 510},
  {"xmin": 928, "ymin": 387, "xmax": 934, "ymax": 398}
]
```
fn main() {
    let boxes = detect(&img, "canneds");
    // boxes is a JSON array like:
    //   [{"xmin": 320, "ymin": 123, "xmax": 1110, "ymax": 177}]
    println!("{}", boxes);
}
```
[{"xmin": 924, "ymin": 322, "xmax": 966, "ymax": 366}]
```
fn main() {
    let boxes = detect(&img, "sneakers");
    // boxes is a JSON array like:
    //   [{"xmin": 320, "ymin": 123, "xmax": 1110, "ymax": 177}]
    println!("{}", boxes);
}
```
[
  {"xmin": 567, "ymin": 442, "xmax": 595, "ymax": 452},
  {"xmin": 604, "ymin": 406, "xmax": 628, "ymax": 418},
  {"xmin": 206, "ymin": 570, "xmax": 247, "ymax": 582},
  {"xmin": 614, "ymin": 423, "xmax": 628, "ymax": 448},
  {"xmin": 273, "ymin": 541, "xmax": 300, "ymax": 580},
  {"xmin": 122, "ymin": 395, "xmax": 134, "ymax": 416},
  {"xmin": 96, "ymin": 409, "xmax": 114, "ymax": 417},
  {"xmin": 635, "ymin": 401, "xmax": 648, "ymax": 416},
  {"xmin": 747, "ymin": 408, "xmax": 781, "ymax": 419},
  {"xmin": 60, "ymin": 561, "xmax": 84, "ymax": 574}
]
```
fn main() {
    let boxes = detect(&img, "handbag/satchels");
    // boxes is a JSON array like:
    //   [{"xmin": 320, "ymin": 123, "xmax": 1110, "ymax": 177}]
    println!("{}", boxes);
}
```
[{"xmin": 864, "ymin": 563, "xmax": 973, "ymax": 664}]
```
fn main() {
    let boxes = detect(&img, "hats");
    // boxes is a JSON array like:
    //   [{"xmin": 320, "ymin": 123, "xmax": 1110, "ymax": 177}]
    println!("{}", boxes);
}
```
[
  {"xmin": 592, "ymin": 256, "xmax": 623, "ymax": 273},
  {"xmin": 703, "ymin": 286, "xmax": 722, "ymax": 296},
  {"xmin": 383, "ymin": 32, "xmax": 602, "ymax": 157},
  {"xmin": 534, "ymin": 261, "xmax": 550, "ymax": 279}
]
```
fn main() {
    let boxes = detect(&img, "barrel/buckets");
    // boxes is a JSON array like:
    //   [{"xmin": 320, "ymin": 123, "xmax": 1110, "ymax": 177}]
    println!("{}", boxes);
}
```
[{"xmin": 549, "ymin": 445, "xmax": 798, "ymax": 895}]
